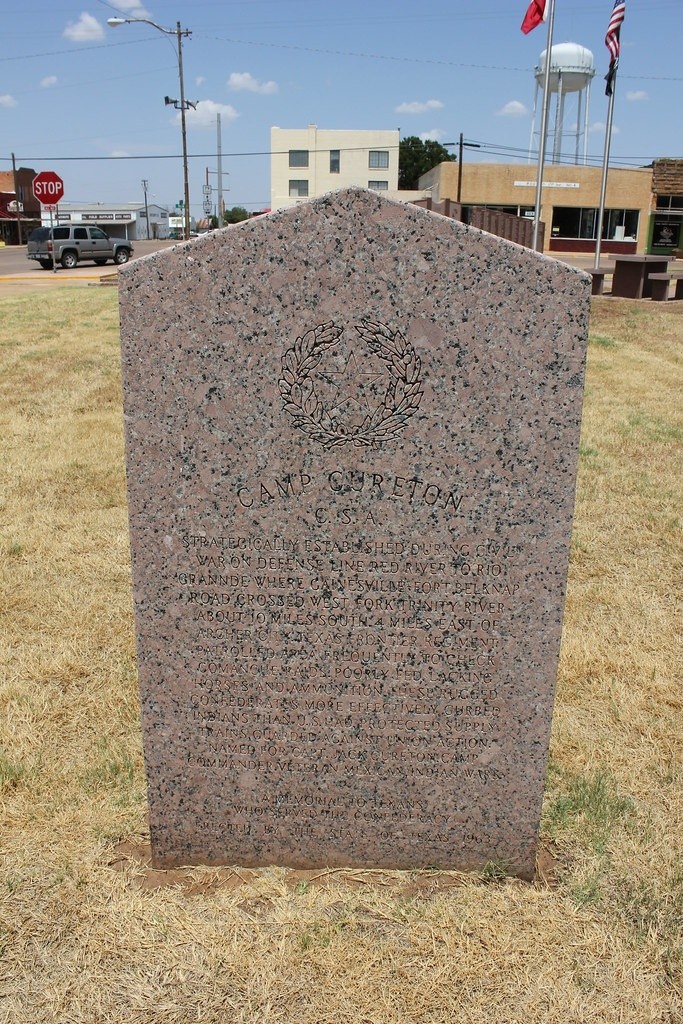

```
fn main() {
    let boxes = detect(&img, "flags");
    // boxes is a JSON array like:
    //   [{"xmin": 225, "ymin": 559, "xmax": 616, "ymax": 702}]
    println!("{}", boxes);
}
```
[
  {"xmin": 520, "ymin": 0, "xmax": 549, "ymax": 35},
  {"xmin": 603, "ymin": 0, "xmax": 626, "ymax": 97}
]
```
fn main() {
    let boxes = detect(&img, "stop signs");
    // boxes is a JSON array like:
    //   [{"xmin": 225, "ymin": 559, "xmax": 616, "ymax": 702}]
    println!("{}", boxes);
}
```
[{"xmin": 32, "ymin": 170, "xmax": 64, "ymax": 206}]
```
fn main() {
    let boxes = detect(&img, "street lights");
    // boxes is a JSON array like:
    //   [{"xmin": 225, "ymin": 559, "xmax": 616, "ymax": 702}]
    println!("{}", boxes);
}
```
[{"xmin": 106, "ymin": 17, "xmax": 192, "ymax": 240}]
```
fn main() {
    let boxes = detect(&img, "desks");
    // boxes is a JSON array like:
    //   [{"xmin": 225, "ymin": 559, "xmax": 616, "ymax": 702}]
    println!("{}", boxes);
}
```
[{"xmin": 608, "ymin": 254, "xmax": 676, "ymax": 299}]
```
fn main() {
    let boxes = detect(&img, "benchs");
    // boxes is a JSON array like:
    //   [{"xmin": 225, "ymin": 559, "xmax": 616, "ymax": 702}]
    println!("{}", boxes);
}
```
[
  {"xmin": 648, "ymin": 273, "xmax": 683, "ymax": 301},
  {"xmin": 584, "ymin": 268, "xmax": 616, "ymax": 295}
]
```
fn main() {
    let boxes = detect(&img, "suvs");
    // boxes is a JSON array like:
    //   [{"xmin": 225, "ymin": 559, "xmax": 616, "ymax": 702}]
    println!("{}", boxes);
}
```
[{"xmin": 26, "ymin": 225, "xmax": 136, "ymax": 270}]
